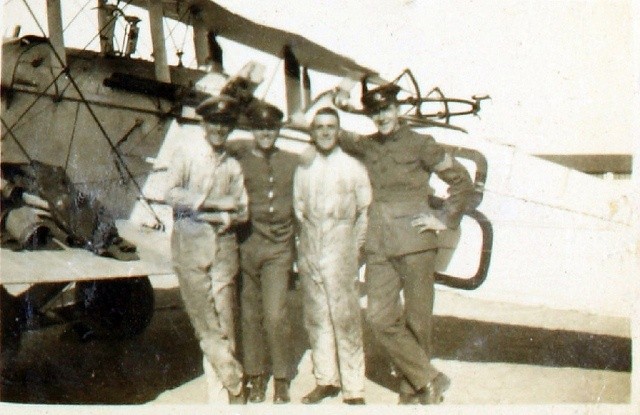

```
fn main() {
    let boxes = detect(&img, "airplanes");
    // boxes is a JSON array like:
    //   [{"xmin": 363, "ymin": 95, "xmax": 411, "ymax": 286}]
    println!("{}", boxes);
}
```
[{"xmin": 0, "ymin": 0, "xmax": 632, "ymax": 355}]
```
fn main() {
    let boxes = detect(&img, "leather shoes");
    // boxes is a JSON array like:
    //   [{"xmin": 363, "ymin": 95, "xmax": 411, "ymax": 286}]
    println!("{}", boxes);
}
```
[
  {"xmin": 274, "ymin": 380, "xmax": 290, "ymax": 403},
  {"xmin": 249, "ymin": 376, "xmax": 265, "ymax": 403},
  {"xmin": 421, "ymin": 372, "xmax": 450, "ymax": 405},
  {"xmin": 170, "ymin": 211, "xmax": 189, "ymax": 219},
  {"xmin": 301, "ymin": 384, "xmax": 340, "ymax": 404},
  {"xmin": 227, "ymin": 388, "xmax": 245, "ymax": 406},
  {"xmin": 398, "ymin": 393, "xmax": 416, "ymax": 406}
]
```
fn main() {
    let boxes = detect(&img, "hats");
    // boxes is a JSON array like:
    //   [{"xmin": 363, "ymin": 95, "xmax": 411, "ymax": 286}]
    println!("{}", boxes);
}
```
[
  {"xmin": 245, "ymin": 103, "xmax": 283, "ymax": 125},
  {"xmin": 361, "ymin": 84, "xmax": 401, "ymax": 111},
  {"xmin": 196, "ymin": 95, "xmax": 240, "ymax": 120}
]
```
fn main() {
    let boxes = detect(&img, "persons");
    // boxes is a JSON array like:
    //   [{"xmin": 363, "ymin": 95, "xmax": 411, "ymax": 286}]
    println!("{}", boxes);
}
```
[
  {"xmin": 354, "ymin": 83, "xmax": 477, "ymax": 406},
  {"xmin": 294, "ymin": 107, "xmax": 372, "ymax": 405},
  {"xmin": 233, "ymin": 102, "xmax": 309, "ymax": 404},
  {"xmin": 164, "ymin": 95, "xmax": 248, "ymax": 402}
]
